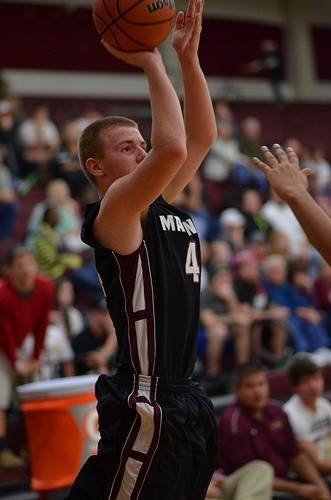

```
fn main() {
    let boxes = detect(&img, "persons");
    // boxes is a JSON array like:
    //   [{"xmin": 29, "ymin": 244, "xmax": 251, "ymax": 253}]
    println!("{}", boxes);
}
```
[{"xmin": 1, "ymin": 1, "xmax": 331, "ymax": 500}]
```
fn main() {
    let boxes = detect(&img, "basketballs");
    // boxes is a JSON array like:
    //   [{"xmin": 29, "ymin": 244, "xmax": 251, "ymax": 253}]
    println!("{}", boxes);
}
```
[{"xmin": 92, "ymin": 0, "xmax": 176, "ymax": 52}]
